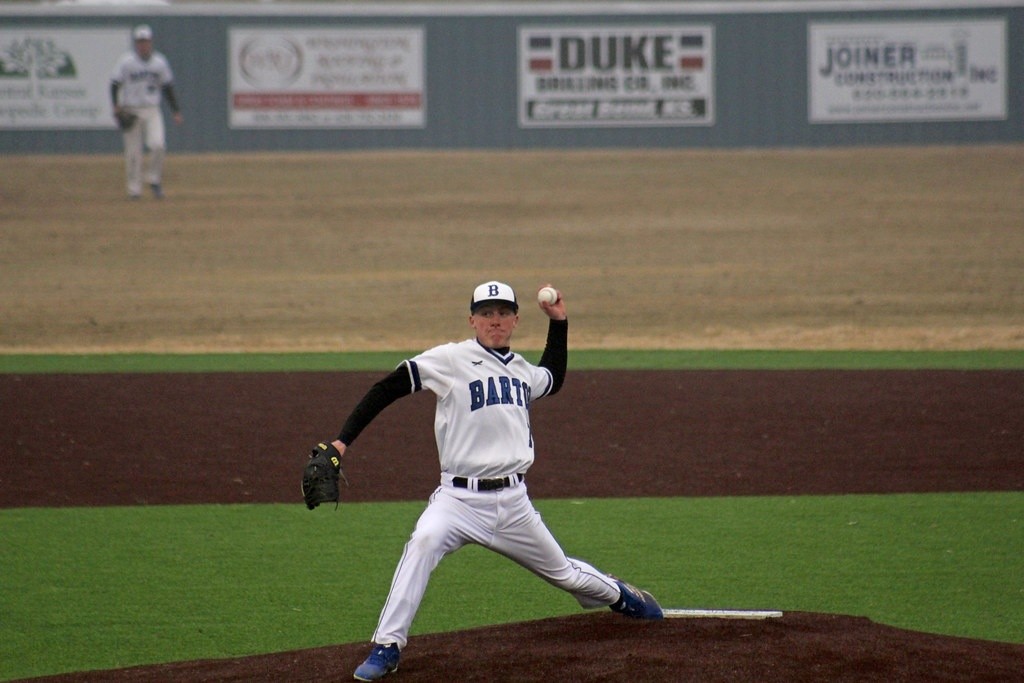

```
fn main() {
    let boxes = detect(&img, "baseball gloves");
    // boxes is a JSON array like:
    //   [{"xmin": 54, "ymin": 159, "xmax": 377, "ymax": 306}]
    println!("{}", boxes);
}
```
[
  {"xmin": 113, "ymin": 111, "xmax": 139, "ymax": 131},
  {"xmin": 301, "ymin": 441, "xmax": 349, "ymax": 511}
]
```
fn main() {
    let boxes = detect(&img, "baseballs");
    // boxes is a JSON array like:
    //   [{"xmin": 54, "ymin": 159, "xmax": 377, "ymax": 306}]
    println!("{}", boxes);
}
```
[{"xmin": 537, "ymin": 287, "xmax": 558, "ymax": 306}]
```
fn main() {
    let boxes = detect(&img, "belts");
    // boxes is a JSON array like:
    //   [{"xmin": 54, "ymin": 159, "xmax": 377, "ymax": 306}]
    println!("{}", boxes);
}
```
[{"xmin": 440, "ymin": 471, "xmax": 526, "ymax": 492}]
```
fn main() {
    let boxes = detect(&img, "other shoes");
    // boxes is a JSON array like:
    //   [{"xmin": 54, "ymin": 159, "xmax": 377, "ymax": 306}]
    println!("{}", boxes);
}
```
[{"xmin": 152, "ymin": 185, "xmax": 164, "ymax": 200}]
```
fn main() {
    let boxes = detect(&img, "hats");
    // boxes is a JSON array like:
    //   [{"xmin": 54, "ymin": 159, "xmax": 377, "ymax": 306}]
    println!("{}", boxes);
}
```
[
  {"xmin": 471, "ymin": 281, "xmax": 519, "ymax": 314},
  {"xmin": 132, "ymin": 23, "xmax": 153, "ymax": 41}
]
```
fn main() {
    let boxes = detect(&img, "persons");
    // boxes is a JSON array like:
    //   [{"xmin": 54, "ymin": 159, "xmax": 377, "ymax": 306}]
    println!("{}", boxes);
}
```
[
  {"xmin": 109, "ymin": 23, "xmax": 182, "ymax": 200},
  {"xmin": 302, "ymin": 280, "xmax": 663, "ymax": 681}
]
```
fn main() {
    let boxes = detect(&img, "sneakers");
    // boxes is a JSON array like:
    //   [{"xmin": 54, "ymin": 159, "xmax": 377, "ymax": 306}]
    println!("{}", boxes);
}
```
[
  {"xmin": 354, "ymin": 642, "xmax": 400, "ymax": 682},
  {"xmin": 607, "ymin": 573, "xmax": 664, "ymax": 619}
]
